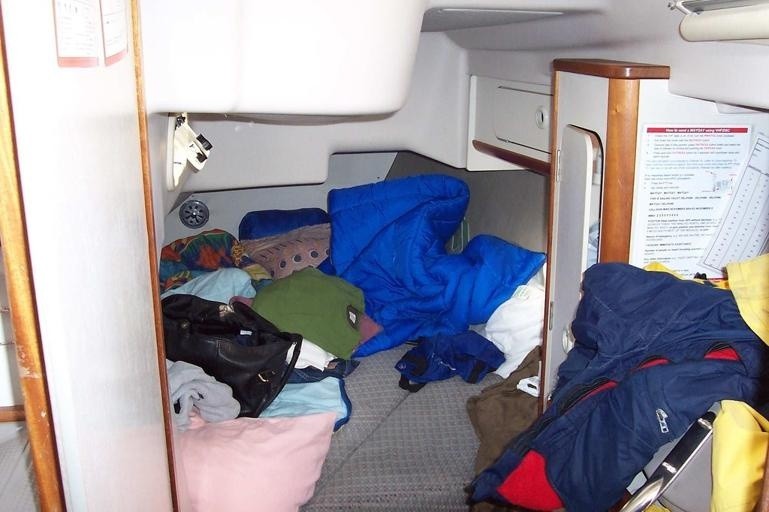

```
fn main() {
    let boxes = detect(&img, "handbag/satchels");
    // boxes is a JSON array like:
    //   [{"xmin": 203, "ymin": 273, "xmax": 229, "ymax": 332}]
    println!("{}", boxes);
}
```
[{"xmin": 161, "ymin": 294, "xmax": 303, "ymax": 417}]
